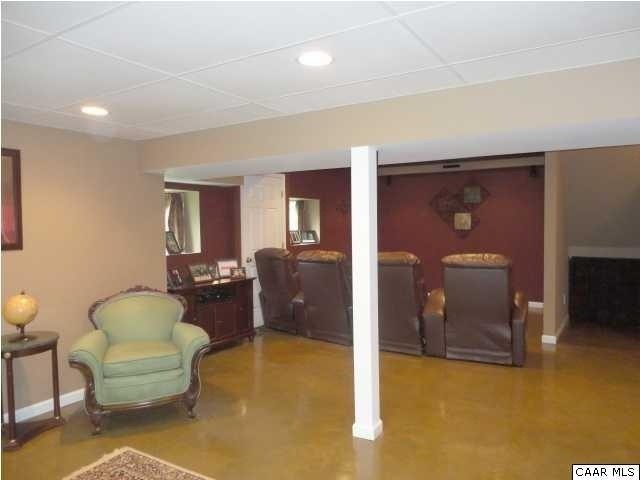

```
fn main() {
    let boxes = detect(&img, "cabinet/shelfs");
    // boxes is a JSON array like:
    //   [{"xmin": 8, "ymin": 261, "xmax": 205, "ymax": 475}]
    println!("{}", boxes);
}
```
[{"xmin": 168, "ymin": 276, "xmax": 256, "ymax": 346}]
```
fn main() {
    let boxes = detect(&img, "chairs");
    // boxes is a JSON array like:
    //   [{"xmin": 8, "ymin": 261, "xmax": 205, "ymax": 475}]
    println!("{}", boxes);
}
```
[
  {"xmin": 68, "ymin": 285, "xmax": 210, "ymax": 435},
  {"xmin": 254, "ymin": 247, "xmax": 528, "ymax": 368}
]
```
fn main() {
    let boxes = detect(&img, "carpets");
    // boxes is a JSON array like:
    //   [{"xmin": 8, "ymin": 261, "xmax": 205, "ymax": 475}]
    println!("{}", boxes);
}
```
[{"xmin": 61, "ymin": 446, "xmax": 215, "ymax": 480}]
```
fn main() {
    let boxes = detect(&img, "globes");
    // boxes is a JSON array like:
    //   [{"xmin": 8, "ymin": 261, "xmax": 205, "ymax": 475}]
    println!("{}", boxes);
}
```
[{"xmin": 3, "ymin": 290, "xmax": 38, "ymax": 343}]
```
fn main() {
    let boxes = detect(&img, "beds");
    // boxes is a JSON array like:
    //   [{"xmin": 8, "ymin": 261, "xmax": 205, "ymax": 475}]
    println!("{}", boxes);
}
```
[{"xmin": 1, "ymin": 330, "xmax": 66, "ymax": 452}]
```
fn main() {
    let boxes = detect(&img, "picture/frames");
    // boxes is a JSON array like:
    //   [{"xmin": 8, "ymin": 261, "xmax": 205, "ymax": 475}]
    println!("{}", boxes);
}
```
[
  {"xmin": 165, "ymin": 231, "xmax": 181, "ymax": 254},
  {"xmin": 169, "ymin": 258, "xmax": 245, "ymax": 288},
  {"xmin": 1, "ymin": 148, "xmax": 24, "ymax": 251}
]
